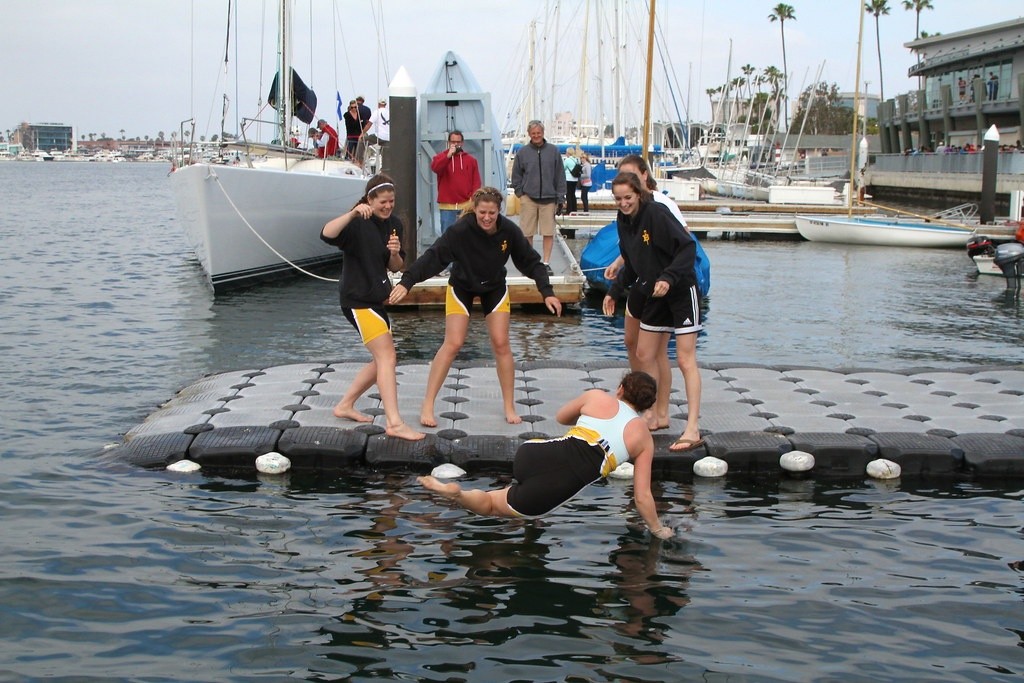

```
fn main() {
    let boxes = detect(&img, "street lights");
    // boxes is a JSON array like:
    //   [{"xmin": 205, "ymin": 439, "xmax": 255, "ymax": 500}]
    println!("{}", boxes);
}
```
[{"xmin": 863, "ymin": 81, "xmax": 872, "ymax": 139}]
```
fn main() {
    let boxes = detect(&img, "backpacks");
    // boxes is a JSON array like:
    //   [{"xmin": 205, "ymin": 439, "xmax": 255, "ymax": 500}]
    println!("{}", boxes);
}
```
[{"xmin": 565, "ymin": 157, "xmax": 583, "ymax": 177}]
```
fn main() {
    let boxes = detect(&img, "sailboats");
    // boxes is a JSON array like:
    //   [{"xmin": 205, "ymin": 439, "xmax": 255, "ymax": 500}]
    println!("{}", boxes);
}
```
[
  {"xmin": 501, "ymin": 0, "xmax": 843, "ymax": 203},
  {"xmin": 13, "ymin": 127, "xmax": 55, "ymax": 161},
  {"xmin": 794, "ymin": 0, "xmax": 973, "ymax": 248},
  {"xmin": 169, "ymin": 0, "xmax": 399, "ymax": 297}
]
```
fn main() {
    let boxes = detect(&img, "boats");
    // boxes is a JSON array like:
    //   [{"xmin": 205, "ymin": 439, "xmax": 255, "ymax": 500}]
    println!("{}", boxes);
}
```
[
  {"xmin": 966, "ymin": 227, "xmax": 1024, "ymax": 278},
  {"xmin": 413, "ymin": 50, "xmax": 506, "ymax": 268},
  {"xmin": 0, "ymin": 148, "xmax": 12, "ymax": 161}
]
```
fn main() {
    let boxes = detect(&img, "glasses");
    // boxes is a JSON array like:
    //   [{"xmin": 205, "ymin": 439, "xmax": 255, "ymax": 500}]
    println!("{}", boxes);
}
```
[{"xmin": 350, "ymin": 106, "xmax": 357, "ymax": 108}]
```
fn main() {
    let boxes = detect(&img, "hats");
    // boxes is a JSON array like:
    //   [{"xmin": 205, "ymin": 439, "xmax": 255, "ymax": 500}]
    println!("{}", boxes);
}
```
[
  {"xmin": 378, "ymin": 97, "xmax": 386, "ymax": 104},
  {"xmin": 317, "ymin": 119, "xmax": 327, "ymax": 127},
  {"xmin": 308, "ymin": 128, "xmax": 317, "ymax": 137}
]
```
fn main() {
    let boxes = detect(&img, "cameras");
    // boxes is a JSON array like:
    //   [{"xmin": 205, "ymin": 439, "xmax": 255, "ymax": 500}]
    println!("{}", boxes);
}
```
[{"xmin": 454, "ymin": 146, "xmax": 462, "ymax": 152}]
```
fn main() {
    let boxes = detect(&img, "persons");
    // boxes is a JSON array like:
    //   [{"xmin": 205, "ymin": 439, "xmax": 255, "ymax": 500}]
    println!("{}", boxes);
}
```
[
  {"xmin": 390, "ymin": 188, "xmax": 562, "ymax": 427},
  {"xmin": 417, "ymin": 371, "xmax": 676, "ymax": 538},
  {"xmin": 321, "ymin": 174, "xmax": 425, "ymax": 439},
  {"xmin": 904, "ymin": 140, "xmax": 1024, "ymax": 156},
  {"xmin": 958, "ymin": 72, "xmax": 999, "ymax": 104},
  {"xmin": 343, "ymin": 96, "xmax": 389, "ymax": 167},
  {"xmin": 564, "ymin": 147, "xmax": 592, "ymax": 215},
  {"xmin": 604, "ymin": 155, "xmax": 690, "ymax": 428},
  {"xmin": 602, "ymin": 175, "xmax": 705, "ymax": 451},
  {"xmin": 431, "ymin": 132, "xmax": 482, "ymax": 276},
  {"xmin": 511, "ymin": 120, "xmax": 568, "ymax": 275},
  {"xmin": 308, "ymin": 120, "xmax": 339, "ymax": 159}
]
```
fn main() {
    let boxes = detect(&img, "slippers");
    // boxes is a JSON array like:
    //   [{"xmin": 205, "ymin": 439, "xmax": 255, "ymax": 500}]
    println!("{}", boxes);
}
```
[
  {"xmin": 669, "ymin": 436, "xmax": 704, "ymax": 451},
  {"xmin": 647, "ymin": 420, "xmax": 669, "ymax": 430}
]
